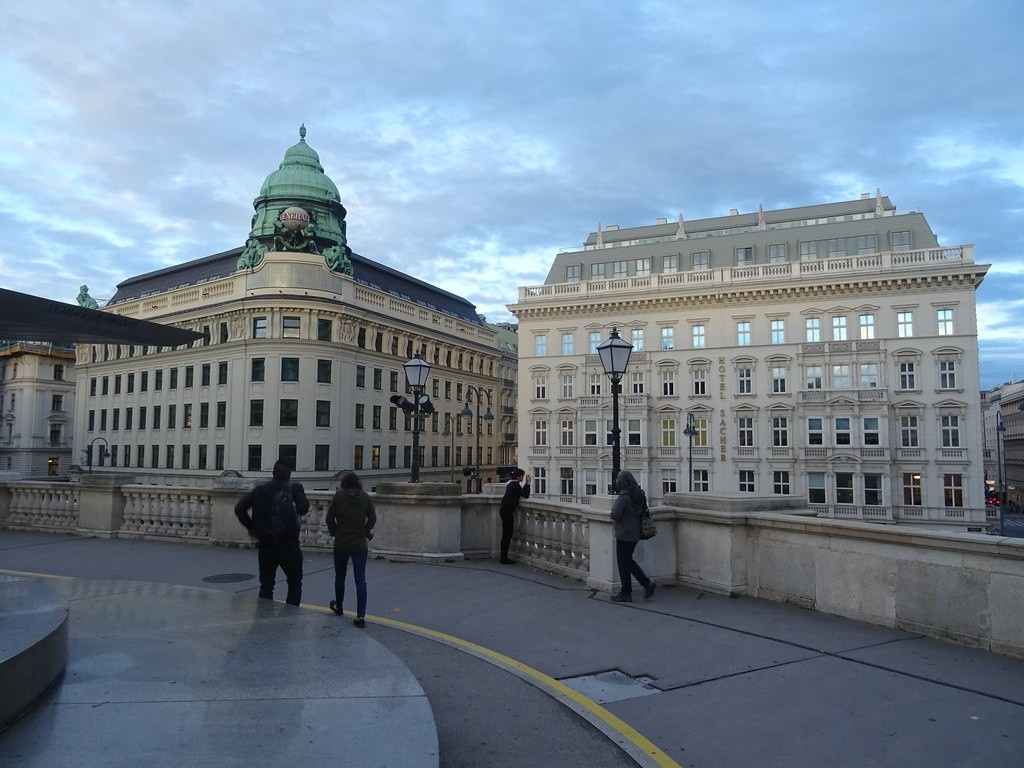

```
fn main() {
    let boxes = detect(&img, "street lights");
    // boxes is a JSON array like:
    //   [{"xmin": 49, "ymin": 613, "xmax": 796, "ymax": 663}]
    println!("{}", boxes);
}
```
[
  {"xmin": 682, "ymin": 412, "xmax": 698, "ymax": 493},
  {"xmin": 596, "ymin": 325, "xmax": 633, "ymax": 492},
  {"xmin": 460, "ymin": 385, "xmax": 495, "ymax": 493},
  {"xmin": 441, "ymin": 416, "xmax": 464, "ymax": 483},
  {"xmin": 89, "ymin": 437, "xmax": 110, "ymax": 473},
  {"xmin": 389, "ymin": 348, "xmax": 434, "ymax": 483},
  {"xmin": 996, "ymin": 410, "xmax": 1007, "ymax": 536}
]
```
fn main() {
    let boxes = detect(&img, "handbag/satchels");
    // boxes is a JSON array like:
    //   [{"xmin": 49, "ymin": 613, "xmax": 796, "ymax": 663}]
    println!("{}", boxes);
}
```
[{"xmin": 639, "ymin": 489, "xmax": 657, "ymax": 540}]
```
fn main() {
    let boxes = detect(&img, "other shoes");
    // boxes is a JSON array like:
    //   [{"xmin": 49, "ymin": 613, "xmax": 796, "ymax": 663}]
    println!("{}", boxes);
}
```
[
  {"xmin": 353, "ymin": 619, "xmax": 365, "ymax": 628},
  {"xmin": 610, "ymin": 591, "xmax": 633, "ymax": 602},
  {"xmin": 644, "ymin": 582, "xmax": 656, "ymax": 598},
  {"xmin": 330, "ymin": 601, "xmax": 344, "ymax": 617},
  {"xmin": 499, "ymin": 558, "xmax": 517, "ymax": 565}
]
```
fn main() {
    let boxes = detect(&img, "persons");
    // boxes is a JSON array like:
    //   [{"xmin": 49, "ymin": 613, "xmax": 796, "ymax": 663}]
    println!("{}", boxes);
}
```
[
  {"xmin": 234, "ymin": 460, "xmax": 310, "ymax": 606},
  {"xmin": 326, "ymin": 473, "xmax": 376, "ymax": 627},
  {"xmin": 499, "ymin": 469, "xmax": 531, "ymax": 564},
  {"xmin": 610, "ymin": 470, "xmax": 657, "ymax": 601}
]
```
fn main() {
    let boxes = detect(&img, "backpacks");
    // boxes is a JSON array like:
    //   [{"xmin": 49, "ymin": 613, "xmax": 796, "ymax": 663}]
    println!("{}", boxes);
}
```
[{"xmin": 264, "ymin": 481, "xmax": 301, "ymax": 534}]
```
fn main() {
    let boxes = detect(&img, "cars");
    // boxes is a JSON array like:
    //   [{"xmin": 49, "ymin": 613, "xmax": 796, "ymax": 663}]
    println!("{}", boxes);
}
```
[{"xmin": 990, "ymin": 499, "xmax": 999, "ymax": 506}]
